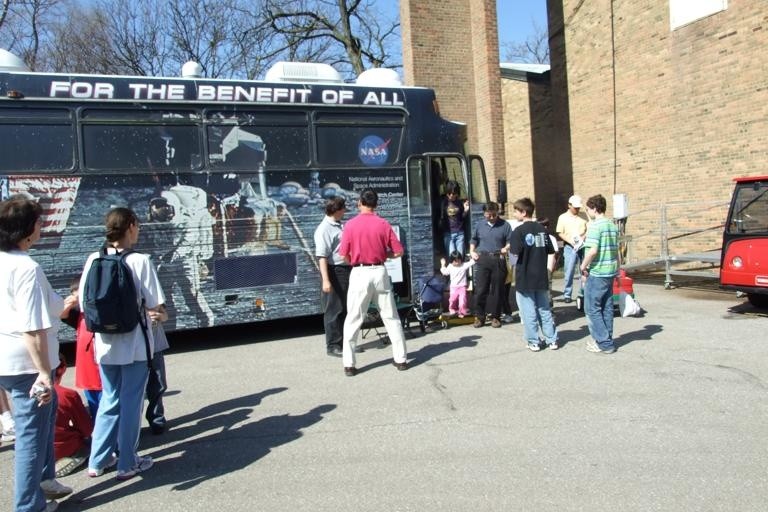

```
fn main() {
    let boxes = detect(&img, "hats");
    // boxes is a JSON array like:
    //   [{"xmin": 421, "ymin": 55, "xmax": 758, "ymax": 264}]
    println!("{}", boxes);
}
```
[{"xmin": 568, "ymin": 195, "xmax": 583, "ymax": 208}]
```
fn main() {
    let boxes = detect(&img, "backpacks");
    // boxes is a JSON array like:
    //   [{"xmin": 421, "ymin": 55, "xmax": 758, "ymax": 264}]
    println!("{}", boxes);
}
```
[{"xmin": 83, "ymin": 244, "xmax": 145, "ymax": 334}]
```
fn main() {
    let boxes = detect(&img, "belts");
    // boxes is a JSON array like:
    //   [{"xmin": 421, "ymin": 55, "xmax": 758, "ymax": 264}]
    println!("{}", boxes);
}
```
[{"xmin": 354, "ymin": 262, "xmax": 384, "ymax": 267}]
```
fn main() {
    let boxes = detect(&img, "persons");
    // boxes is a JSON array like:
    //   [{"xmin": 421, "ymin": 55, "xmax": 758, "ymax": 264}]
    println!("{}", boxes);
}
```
[
  {"xmin": 148, "ymin": 191, "xmax": 214, "ymax": 332},
  {"xmin": 337, "ymin": 188, "xmax": 408, "ymax": 377},
  {"xmin": 313, "ymin": 197, "xmax": 364, "ymax": 359},
  {"xmin": 436, "ymin": 181, "xmax": 560, "ymax": 352},
  {"xmin": 580, "ymin": 195, "xmax": 620, "ymax": 354},
  {"xmin": 555, "ymin": 196, "xmax": 589, "ymax": 303},
  {"xmin": 1, "ymin": 193, "xmax": 171, "ymax": 512}
]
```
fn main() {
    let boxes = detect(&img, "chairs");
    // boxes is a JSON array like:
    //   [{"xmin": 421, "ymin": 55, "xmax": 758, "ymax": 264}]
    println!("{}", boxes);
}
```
[{"xmin": 361, "ymin": 293, "xmax": 416, "ymax": 345}]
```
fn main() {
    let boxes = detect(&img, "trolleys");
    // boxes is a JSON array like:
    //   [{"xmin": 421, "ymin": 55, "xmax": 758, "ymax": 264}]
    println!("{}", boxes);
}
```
[{"xmin": 577, "ymin": 240, "xmax": 641, "ymax": 314}]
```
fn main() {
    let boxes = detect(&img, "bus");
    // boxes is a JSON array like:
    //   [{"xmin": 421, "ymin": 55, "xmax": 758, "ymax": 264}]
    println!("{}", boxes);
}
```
[{"xmin": 0, "ymin": 45, "xmax": 509, "ymax": 341}]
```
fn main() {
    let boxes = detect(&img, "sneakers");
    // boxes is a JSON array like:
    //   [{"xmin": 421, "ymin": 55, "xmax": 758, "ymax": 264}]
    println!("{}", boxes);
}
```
[
  {"xmin": 549, "ymin": 342, "xmax": 558, "ymax": 350},
  {"xmin": 355, "ymin": 346, "xmax": 363, "ymax": 352},
  {"xmin": 88, "ymin": 452, "xmax": 117, "ymax": 476},
  {"xmin": 55, "ymin": 456, "xmax": 89, "ymax": 477},
  {"xmin": 40, "ymin": 500, "xmax": 58, "ymax": 512},
  {"xmin": 116, "ymin": 456, "xmax": 154, "ymax": 480},
  {"xmin": 448, "ymin": 309, "xmax": 513, "ymax": 328},
  {"xmin": 1, "ymin": 422, "xmax": 18, "ymax": 441},
  {"xmin": 327, "ymin": 348, "xmax": 342, "ymax": 357},
  {"xmin": 344, "ymin": 367, "xmax": 357, "ymax": 376},
  {"xmin": 565, "ymin": 297, "xmax": 571, "ymax": 302},
  {"xmin": 586, "ymin": 340, "xmax": 616, "ymax": 354},
  {"xmin": 40, "ymin": 478, "xmax": 73, "ymax": 500},
  {"xmin": 392, "ymin": 359, "xmax": 408, "ymax": 371},
  {"xmin": 526, "ymin": 341, "xmax": 540, "ymax": 352}
]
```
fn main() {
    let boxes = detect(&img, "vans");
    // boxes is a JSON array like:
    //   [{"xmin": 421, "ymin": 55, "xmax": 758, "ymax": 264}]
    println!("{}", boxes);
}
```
[{"xmin": 719, "ymin": 173, "xmax": 768, "ymax": 304}]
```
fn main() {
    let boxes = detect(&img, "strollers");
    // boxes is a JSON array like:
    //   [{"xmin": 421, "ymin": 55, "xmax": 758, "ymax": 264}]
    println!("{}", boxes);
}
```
[{"xmin": 401, "ymin": 274, "xmax": 447, "ymax": 336}]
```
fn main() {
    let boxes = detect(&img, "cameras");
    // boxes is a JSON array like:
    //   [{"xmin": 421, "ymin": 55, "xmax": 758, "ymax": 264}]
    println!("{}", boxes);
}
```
[{"xmin": 32, "ymin": 384, "xmax": 47, "ymax": 398}]
